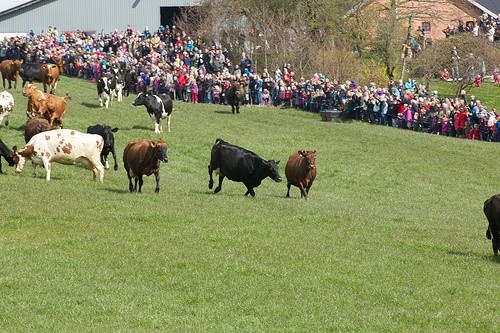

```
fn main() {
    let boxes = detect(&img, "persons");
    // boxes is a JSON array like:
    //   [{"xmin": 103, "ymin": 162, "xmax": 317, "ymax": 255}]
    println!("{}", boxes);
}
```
[{"xmin": 0, "ymin": 13, "xmax": 500, "ymax": 147}]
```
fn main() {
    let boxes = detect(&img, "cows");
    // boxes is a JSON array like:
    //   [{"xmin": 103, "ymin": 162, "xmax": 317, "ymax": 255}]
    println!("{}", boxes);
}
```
[
  {"xmin": 97, "ymin": 67, "xmax": 124, "ymax": 105},
  {"xmin": 87, "ymin": 125, "xmax": 118, "ymax": 171},
  {"xmin": 122, "ymin": 136, "xmax": 169, "ymax": 194},
  {"xmin": 482, "ymin": 194, "xmax": 500, "ymax": 257},
  {"xmin": 208, "ymin": 138, "xmax": 283, "ymax": 200},
  {"xmin": 0, "ymin": 90, "xmax": 15, "ymax": 130},
  {"xmin": 0, "ymin": 58, "xmax": 23, "ymax": 90},
  {"xmin": 41, "ymin": 63, "xmax": 59, "ymax": 94},
  {"xmin": 23, "ymin": 82, "xmax": 72, "ymax": 130},
  {"xmin": 13, "ymin": 129, "xmax": 106, "ymax": 184},
  {"xmin": 19, "ymin": 64, "xmax": 41, "ymax": 89},
  {"xmin": 23, "ymin": 116, "xmax": 57, "ymax": 147},
  {"xmin": 225, "ymin": 79, "xmax": 247, "ymax": 114},
  {"xmin": 0, "ymin": 138, "xmax": 19, "ymax": 175},
  {"xmin": 284, "ymin": 149, "xmax": 318, "ymax": 202},
  {"xmin": 132, "ymin": 90, "xmax": 174, "ymax": 133}
]
